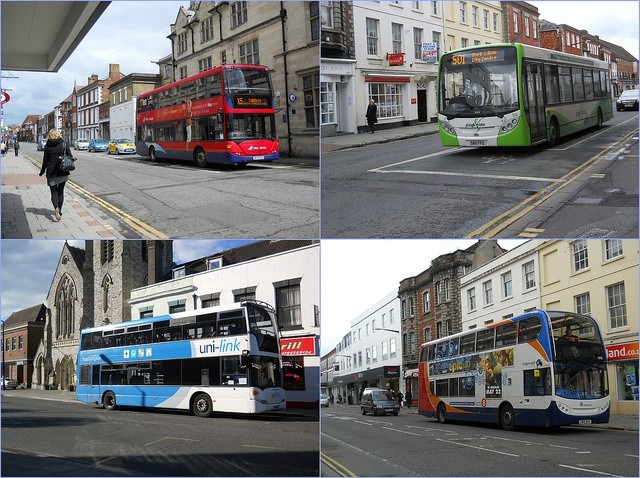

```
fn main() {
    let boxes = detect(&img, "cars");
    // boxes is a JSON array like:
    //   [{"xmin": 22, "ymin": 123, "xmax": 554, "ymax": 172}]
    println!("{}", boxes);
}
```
[
  {"xmin": 74, "ymin": 137, "xmax": 90, "ymax": 151},
  {"xmin": 37, "ymin": 139, "xmax": 48, "ymax": 151},
  {"xmin": 107, "ymin": 137, "xmax": 137, "ymax": 155},
  {"xmin": 320, "ymin": 393, "xmax": 329, "ymax": 407},
  {"xmin": 0, "ymin": 378, "xmax": 18, "ymax": 390},
  {"xmin": 87, "ymin": 138, "xmax": 109, "ymax": 153},
  {"xmin": 616, "ymin": 89, "xmax": 640, "ymax": 112}
]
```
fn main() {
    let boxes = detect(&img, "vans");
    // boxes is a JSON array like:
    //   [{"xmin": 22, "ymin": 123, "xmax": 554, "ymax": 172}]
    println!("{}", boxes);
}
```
[{"xmin": 360, "ymin": 387, "xmax": 400, "ymax": 416}]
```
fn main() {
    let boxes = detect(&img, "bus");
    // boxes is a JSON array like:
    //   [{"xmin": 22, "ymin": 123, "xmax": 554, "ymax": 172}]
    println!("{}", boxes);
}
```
[
  {"xmin": 135, "ymin": 63, "xmax": 287, "ymax": 169},
  {"xmin": 435, "ymin": 42, "xmax": 614, "ymax": 148},
  {"xmin": 417, "ymin": 308, "xmax": 611, "ymax": 431},
  {"xmin": 75, "ymin": 299, "xmax": 287, "ymax": 419}
]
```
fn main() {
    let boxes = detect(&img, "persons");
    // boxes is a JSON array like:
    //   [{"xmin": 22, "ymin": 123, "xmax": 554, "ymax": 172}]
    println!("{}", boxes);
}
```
[
  {"xmin": 38, "ymin": 128, "xmax": 75, "ymax": 220},
  {"xmin": 14, "ymin": 140, "xmax": 19, "ymax": 156},
  {"xmin": 397, "ymin": 390, "xmax": 403, "ymax": 408},
  {"xmin": 365, "ymin": 99, "xmax": 378, "ymax": 134},
  {"xmin": 180, "ymin": 97, "xmax": 193, "ymax": 151},
  {"xmin": 1, "ymin": 141, "xmax": 6, "ymax": 157},
  {"xmin": 336, "ymin": 395, "xmax": 342, "ymax": 405},
  {"xmin": 405, "ymin": 388, "xmax": 412, "ymax": 408}
]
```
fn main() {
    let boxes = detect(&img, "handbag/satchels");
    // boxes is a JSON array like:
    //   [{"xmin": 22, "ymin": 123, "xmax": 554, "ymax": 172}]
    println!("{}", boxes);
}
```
[{"xmin": 56, "ymin": 155, "xmax": 77, "ymax": 171}]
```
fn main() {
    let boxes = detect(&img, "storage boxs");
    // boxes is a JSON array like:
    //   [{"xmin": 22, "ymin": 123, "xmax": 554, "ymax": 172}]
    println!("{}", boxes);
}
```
[{"xmin": 110, "ymin": 338, "xmax": 115, "ymax": 346}]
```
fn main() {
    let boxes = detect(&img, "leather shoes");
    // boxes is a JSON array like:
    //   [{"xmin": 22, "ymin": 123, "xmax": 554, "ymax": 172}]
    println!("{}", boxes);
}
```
[{"xmin": 370, "ymin": 131, "xmax": 374, "ymax": 134}]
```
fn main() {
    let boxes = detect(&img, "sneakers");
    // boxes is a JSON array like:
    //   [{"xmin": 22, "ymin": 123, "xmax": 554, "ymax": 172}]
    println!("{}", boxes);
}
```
[
  {"xmin": 59, "ymin": 208, "xmax": 63, "ymax": 216},
  {"xmin": 55, "ymin": 207, "xmax": 61, "ymax": 220}
]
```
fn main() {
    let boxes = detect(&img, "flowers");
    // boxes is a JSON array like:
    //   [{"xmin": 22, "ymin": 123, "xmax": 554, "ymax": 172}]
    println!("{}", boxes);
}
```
[{"xmin": 67, "ymin": 383, "xmax": 73, "ymax": 386}]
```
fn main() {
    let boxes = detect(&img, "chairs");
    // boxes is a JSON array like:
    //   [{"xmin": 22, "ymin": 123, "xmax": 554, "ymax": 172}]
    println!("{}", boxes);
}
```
[
  {"xmin": 545, "ymin": 379, "xmax": 551, "ymax": 394},
  {"xmin": 110, "ymin": 339, "xmax": 115, "ymax": 346},
  {"xmin": 473, "ymin": 87, "xmax": 485, "ymax": 105},
  {"xmin": 156, "ymin": 334, "xmax": 161, "ymax": 341}
]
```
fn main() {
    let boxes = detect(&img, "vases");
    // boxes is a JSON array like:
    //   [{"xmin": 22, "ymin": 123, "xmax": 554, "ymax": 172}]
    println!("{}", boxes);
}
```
[{"xmin": 67, "ymin": 386, "xmax": 74, "ymax": 391}]
